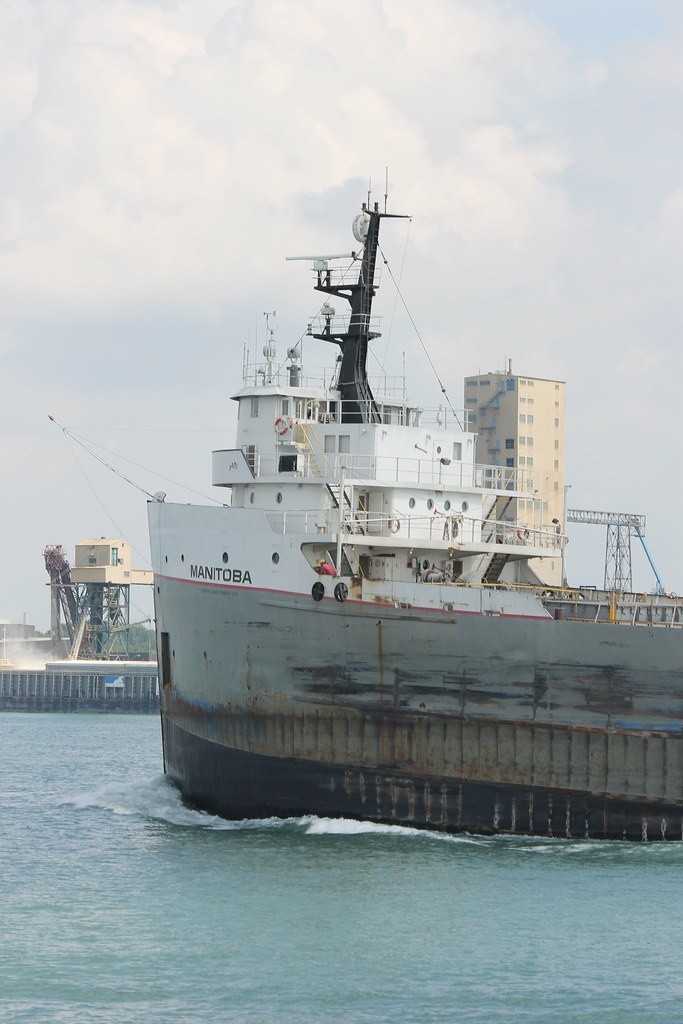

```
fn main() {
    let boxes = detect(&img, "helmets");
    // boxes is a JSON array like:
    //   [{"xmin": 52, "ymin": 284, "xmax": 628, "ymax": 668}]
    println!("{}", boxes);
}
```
[{"xmin": 320, "ymin": 559, "xmax": 326, "ymax": 564}]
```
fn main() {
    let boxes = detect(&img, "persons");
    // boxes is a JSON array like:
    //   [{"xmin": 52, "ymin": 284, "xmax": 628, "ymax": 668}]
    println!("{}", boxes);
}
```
[{"xmin": 319, "ymin": 559, "xmax": 337, "ymax": 575}]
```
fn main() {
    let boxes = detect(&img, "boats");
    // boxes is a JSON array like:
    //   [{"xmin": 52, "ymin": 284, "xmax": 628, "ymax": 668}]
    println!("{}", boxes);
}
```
[{"xmin": 45, "ymin": 165, "xmax": 683, "ymax": 845}]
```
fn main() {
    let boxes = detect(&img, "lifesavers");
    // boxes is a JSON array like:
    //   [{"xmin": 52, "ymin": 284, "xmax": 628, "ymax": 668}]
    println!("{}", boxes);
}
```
[
  {"xmin": 386, "ymin": 513, "xmax": 401, "ymax": 533},
  {"xmin": 516, "ymin": 525, "xmax": 530, "ymax": 542},
  {"xmin": 273, "ymin": 416, "xmax": 292, "ymax": 435}
]
